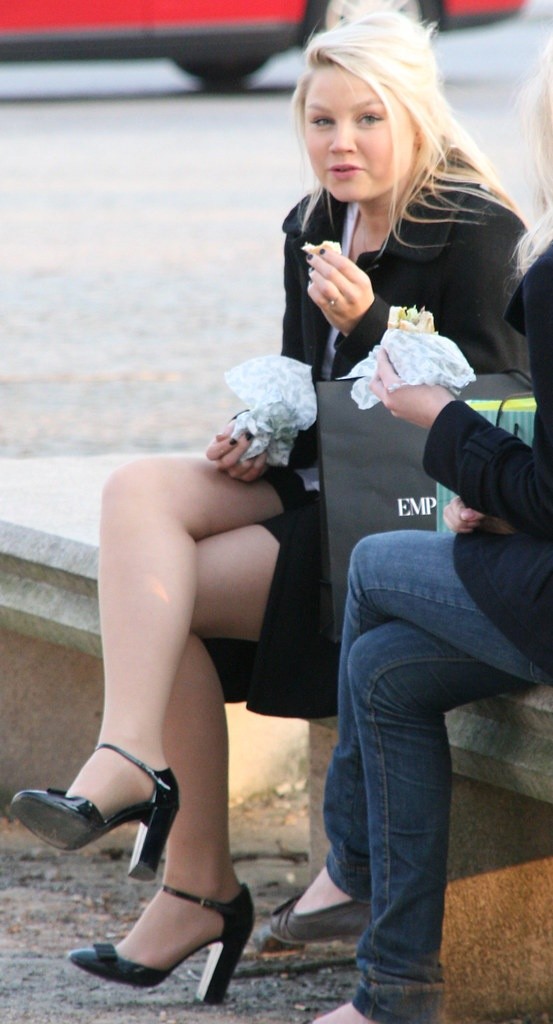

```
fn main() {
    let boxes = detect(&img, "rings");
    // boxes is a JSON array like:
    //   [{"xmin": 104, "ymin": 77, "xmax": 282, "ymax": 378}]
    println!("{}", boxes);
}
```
[{"xmin": 328, "ymin": 298, "xmax": 338, "ymax": 307}]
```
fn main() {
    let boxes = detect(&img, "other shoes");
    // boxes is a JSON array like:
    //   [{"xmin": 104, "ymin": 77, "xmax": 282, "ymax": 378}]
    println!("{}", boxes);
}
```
[
  {"xmin": 269, "ymin": 879, "xmax": 373, "ymax": 942},
  {"xmin": 302, "ymin": 1000, "xmax": 379, "ymax": 1024}
]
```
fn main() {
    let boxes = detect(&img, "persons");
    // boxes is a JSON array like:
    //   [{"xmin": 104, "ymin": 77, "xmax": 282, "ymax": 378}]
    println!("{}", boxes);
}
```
[{"xmin": 10, "ymin": 12, "xmax": 553, "ymax": 1024}]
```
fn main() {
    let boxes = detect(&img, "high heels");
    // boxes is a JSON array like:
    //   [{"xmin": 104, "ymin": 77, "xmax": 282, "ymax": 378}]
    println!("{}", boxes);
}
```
[
  {"xmin": 70, "ymin": 883, "xmax": 254, "ymax": 1005},
  {"xmin": 12, "ymin": 743, "xmax": 179, "ymax": 879}
]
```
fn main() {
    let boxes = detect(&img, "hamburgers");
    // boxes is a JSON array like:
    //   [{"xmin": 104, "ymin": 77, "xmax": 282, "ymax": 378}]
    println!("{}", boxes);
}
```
[{"xmin": 387, "ymin": 306, "xmax": 435, "ymax": 334}]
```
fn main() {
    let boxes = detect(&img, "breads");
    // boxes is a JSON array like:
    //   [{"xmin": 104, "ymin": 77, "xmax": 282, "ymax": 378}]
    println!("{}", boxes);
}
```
[{"xmin": 302, "ymin": 240, "xmax": 342, "ymax": 255}]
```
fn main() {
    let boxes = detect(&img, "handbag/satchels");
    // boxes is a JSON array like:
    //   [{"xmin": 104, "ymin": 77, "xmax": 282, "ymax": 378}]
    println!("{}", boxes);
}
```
[
  {"xmin": 437, "ymin": 391, "xmax": 538, "ymax": 534},
  {"xmin": 317, "ymin": 368, "xmax": 544, "ymax": 643}
]
